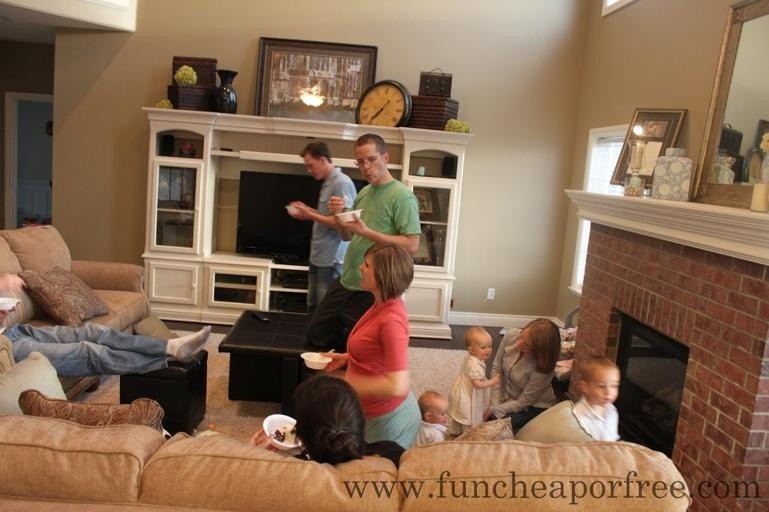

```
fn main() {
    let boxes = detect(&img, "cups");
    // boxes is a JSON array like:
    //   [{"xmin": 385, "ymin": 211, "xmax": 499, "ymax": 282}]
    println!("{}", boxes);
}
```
[{"xmin": 417, "ymin": 165, "xmax": 425, "ymax": 175}]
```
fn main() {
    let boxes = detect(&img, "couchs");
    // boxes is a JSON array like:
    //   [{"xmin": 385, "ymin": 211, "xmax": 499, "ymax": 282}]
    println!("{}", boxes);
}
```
[
  {"xmin": 0, "ymin": 405, "xmax": 693, "ymax": 512},
  {"xmin": 0, "ymin": 223, "xmax": 151, "ymax": 405}
]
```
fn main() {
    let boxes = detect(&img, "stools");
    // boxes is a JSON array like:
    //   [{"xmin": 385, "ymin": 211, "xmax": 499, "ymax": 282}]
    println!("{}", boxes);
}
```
[{"xmin": 120, "ymin": 346, "xmax": 211, "ymax": 435}]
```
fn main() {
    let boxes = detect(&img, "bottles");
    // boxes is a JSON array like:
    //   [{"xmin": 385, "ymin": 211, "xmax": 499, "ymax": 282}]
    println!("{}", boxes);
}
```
[
  {"xmin": 713, "ymin": 154, "xmax": 737, "ymax": 186},
  {"xmin": 654, "ymin": 147, "xmax": 692, "ymax": 204}
]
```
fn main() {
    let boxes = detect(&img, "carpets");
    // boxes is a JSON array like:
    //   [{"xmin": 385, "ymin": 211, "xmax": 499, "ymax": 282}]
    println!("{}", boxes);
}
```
[{"xmin": 67, "ymin": 325, "xmax": 492, "ymax": 446}]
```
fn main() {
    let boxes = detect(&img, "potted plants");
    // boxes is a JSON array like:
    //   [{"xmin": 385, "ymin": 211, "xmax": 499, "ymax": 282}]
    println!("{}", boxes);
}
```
[{"xmin": 170, "ymin": 63, "xmax": 207, "ymax": 112}]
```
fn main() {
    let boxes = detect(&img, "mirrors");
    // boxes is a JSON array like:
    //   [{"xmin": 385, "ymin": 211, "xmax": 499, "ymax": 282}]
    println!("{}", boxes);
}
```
[{"xmin": 690, "ymin": 1, "xmax": 769, "ymax": 212}]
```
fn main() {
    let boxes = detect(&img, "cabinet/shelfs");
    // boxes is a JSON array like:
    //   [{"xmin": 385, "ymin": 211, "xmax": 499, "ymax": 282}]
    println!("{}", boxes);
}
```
[{"xmin": 138, "ymin": 102, "xmax": 473, "ymax": 342}]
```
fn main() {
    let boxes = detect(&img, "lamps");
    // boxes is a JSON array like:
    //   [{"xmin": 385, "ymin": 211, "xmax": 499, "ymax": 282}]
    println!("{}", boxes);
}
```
[{"xmin": 621, "ymin": 124, "xmax": 649, "ymax": 197}]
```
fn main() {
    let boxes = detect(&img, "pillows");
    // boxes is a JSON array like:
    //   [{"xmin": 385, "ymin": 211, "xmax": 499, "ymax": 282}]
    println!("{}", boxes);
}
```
[
  {"xmin": 133, "ymin": 315, "xmax": 180, "ymax": 342},
  {"xmin": 0, "ymin": 348, "xmax": 68, "ymax": 416},
  {"xmin": 17, "ymin": 264, "xmax": 108, "ymax": 327},
  {"xmin": 17, "ymin": 390, "xmax": 170, "ymax": 439}
]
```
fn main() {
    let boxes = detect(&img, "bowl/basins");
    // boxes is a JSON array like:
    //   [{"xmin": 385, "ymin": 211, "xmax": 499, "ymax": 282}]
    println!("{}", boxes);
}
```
[
  {"xmin": 263, "ymin": 413, "xmax": 305, "ymax": 452},
  {"xmin": 298, "ymin": 351, "xmax": 333, "ymax": 370},
  {"xmin": 336, "ymin": 208, "xmax": 365, "ymax": 224}
]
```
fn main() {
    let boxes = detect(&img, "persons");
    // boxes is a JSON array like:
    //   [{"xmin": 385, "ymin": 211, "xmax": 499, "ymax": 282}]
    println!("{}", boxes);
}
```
[
  {"xmin": 309, "ymin": 133, "xmax": 421, "ymax": 354},
  {"xmin": 448, "ymin": 326, "xmax": 499, "ymax": 437},
  {"xmin": 249, "ymin": 374, "xmax": 405, "ymax": 469},
  {"xmin": 482, "ymin": 317, "xmax": 561, "ymax": 430},
  {"xmin": 572, "ymin": 355, "xmax": 622, "ymax": 442},
  {"xmin": 287, "ymin": 144, "xmax": 357, "ymax": 314},
  {"xmin": 416, "ymin": 391, "xmax": 449, "ymax": 447},
  {"xmin": 319, "ymin": 242, "xmax": 421, "ymax": 450},
  {"xmin": 0, "ymin": 275, "xmax": 211, "ymax": 376}
]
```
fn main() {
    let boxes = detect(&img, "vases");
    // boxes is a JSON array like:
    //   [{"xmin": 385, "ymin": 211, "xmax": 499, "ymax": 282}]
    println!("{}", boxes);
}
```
[
  {"xmin": 209, "ymin": 67, "xmax": 240, "ymax": 115},
  {"xmin": 651, "ymin": 147, "xmax": 696, "ymax": 203},
  {"xmin": 411, "ymin": 93, "xmax": 460, "ymax": 131}
]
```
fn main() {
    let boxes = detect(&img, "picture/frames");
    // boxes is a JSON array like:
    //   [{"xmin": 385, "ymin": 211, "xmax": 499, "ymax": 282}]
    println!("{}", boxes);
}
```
[
  {"xmin": 413, "ymin": 187, "xmax": 443, "ymax": 221},
  {"xmin": 251, "ymin": 35, "xmax": 379, "ymax": 127},
  {"xmin": 411, "ymin": 222, "xmax": 438, "ymax": 267},
  {"xmin": 608, "ymin": 108, "xmax": 687, "ymax": 193}
]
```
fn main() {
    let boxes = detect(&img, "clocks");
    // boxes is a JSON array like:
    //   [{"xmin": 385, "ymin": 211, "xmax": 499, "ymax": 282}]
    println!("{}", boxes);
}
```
[{"xmin": 354, "ymin": 78, "xmax": 412, "ymax": 128}]
